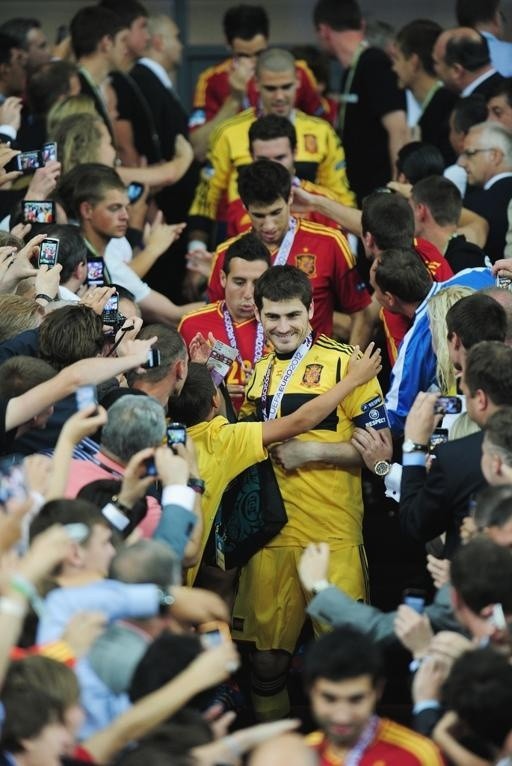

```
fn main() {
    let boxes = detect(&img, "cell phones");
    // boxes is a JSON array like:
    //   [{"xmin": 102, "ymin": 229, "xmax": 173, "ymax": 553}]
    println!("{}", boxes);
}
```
[
  {"xmin": 198, "ymin": 621, "xmax": 231, "ymax": 648},
  {"xmin": 56, "ymin": 25, "xmax": 64, "ymax": 43},
  {"xmin": 16, "ymin": 151, "xmax": 38, "ymax": 171},
  {"xmin": 429, "ymin": 428, "xmax": 448, "ymax": 456},
  {"xmin": 141, "ymin": 350, "xmax": 161, "ymax": 368},
  {"xmin": 497, "ymin": 275, "xmax": 511, "ymax": 290},
  {"xmin": 76, "ymin": 382, "xmax": 97, "ymax": 413},
  {"xmin": 40, "ymin": 142, "xmax": 57, "ymax": 167},
  {"xmin": 405, "ymin": 589, "xmax": 424, "ymax": 612},
  {"xmin": 37, "ymin": 237, "xmax": 119, "ymax": 312},
  {"xmin": 146, "ymin": 458, "xmax": 157, "ymax": 475},
  {"xmin": 433, "ymin": 396, "xmax": 461, "ymax": 414},
  {"xmin": 21, "ymin": 200, "xmax": 56, "ymax": 226},
  {"xmin": 125, "ymin": 181, "xmax": 143, "ymax": 204},
  {"xmin": 167, "ymin": 423, "xmax": 186, "ymax": 451}
]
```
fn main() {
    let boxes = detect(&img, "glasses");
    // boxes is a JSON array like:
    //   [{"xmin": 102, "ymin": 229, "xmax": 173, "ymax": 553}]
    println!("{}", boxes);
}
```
[{"xmin": 463, "ymin": 148, "xmax": 504, "ymax": 155}]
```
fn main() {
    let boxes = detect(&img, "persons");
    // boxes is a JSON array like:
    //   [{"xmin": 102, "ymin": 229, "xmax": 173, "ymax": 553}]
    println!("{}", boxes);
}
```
[{"xmin": 1, "ymin": 2, "xmax": 511, "ymax": 766}]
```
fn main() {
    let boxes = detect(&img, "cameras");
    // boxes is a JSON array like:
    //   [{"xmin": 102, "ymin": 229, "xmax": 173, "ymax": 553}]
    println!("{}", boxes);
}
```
[{"xmin": 100, "ymin": 313, "xmax": 128, "ymax": 338}]
